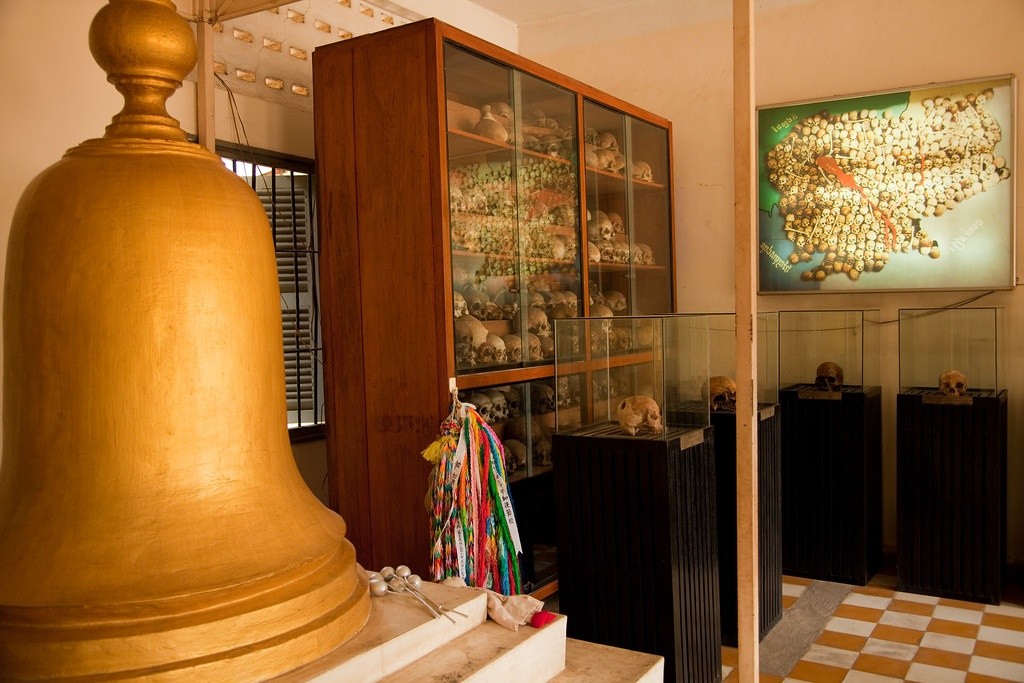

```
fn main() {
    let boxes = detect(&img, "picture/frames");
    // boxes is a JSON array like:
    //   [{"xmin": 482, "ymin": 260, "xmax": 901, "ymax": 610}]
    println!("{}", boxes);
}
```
[{"xmin": 757, "ymin": 73, "xmax": 1016, "ymax": 296}]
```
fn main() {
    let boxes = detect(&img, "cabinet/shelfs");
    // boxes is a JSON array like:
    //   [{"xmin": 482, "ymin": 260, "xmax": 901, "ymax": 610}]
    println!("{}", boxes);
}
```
[{"xmin": 309, "ymin": 18, "xmax": 682, "ymax": 603}]
